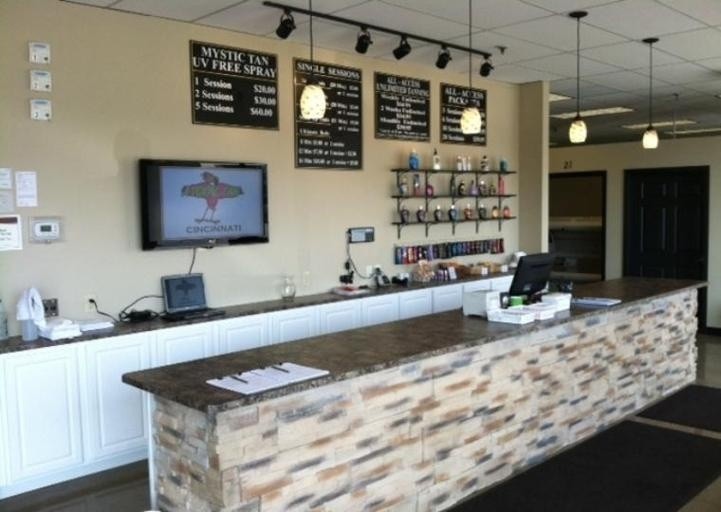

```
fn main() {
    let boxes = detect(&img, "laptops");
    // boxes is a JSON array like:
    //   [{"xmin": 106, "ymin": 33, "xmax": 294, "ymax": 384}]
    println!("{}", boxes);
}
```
[{"xmin": 160, "ymin": 272, "xmax": 225, "ymax": 322}]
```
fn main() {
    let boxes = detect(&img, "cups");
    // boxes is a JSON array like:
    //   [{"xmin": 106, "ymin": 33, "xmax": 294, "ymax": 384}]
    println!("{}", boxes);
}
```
[{"xmin": 510, "ymin": 296, "xmax": 523, "ymax": 305}]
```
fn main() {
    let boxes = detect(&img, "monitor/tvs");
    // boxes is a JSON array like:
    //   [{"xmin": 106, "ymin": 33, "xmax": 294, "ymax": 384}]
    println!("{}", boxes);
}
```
[
  {"xmin": 138, "ymin": 156, "xmax": 270, "ymax": 249},
  {"xmin": 509, "ymin": 251, "xmax": 557, "ymax": 302}
]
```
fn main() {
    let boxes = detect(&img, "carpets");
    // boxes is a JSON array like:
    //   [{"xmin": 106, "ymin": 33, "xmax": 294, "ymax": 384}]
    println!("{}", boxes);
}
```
[
  {"xmin": 636, "ymin": 385, "xmax": 720, "ymax": 432},
  {"xmin": 442, "ymin": 420, "xmax": 721, "ymax": 512}
]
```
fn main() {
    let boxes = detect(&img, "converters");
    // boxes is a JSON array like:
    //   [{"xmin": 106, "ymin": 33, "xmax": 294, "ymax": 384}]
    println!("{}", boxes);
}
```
[{"xmin": 127, "ymin": 311, "xmax": 151, "ymax": 322}]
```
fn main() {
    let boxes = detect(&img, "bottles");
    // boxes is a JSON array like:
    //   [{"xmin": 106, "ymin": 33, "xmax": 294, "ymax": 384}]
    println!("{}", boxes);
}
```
[
  {"xmin": 281, "ymin": 273, "xmax": 296, "ymax": 303},
  {"xmin": 401, "ymin": 203, "xmax": 512, "ymax": 222},
  {"xmin": 433, "ymin": 147, "xmax": 440, "ymax": 170},
  {"xmin": 500, "ymin": 156, "xmax": 508, "ymax": 171},
  {"xmin": 456, "ymin": 155, "xmax": 473, "ymax": 170},
  {"xmin": 437, "ymin": 265, "xmax": 449, "ymax": 281},
  {"xmin": 395, "ymin": 238, "xmax": 505, "ymax": 264},
  {"xmin": 399, "ymin": 172, "xmax": 505, "ymax": 196},
  {"xmin": 409, "ymin": 147, "xmax": 419, "ymax": 170},
  {"xmin": 479, "ymin": 156, "xmax": 488, "ymax": 171},
  {"xmin": 0, "ymin": 296, "xmax": 8, "ymax": 340},
  {"xmin": 22, "ymin": 290, "xmax": 39, "ymax": 341}
]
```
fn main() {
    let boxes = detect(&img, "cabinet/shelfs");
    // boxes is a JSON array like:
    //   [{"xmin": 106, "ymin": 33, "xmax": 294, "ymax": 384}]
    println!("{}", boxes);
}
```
[
  {"xmin": 492, "ymin": 269, "xmax": 514, "ymax": 294},
  {"xmin": 364, "ymin": 284, "xmax": 433, "ymax": 325},
  {"xmin": 0, "ymin": 315, "xmax": 154, "ymax": 499},
  {"xmin": 153, "ymin": 301, "xmax": 273, "ymax": 366},
  {"xmin": 391, "ymin": 165, "xmax": 517, "ymax": 239},
  {"xmin": 433, "ymin": 273, "xmax": 492, "ymax": 312},
  {"xmin": 272, "ymin": 291, "xmax": 364, "ymax": 344}
]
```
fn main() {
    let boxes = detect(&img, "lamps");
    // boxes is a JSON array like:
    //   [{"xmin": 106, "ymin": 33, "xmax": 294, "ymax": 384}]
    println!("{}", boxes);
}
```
[
  {"xmin": 459, "ymin": 0, "xmax": 482, "ymax": 135},
  {"xmin": 261, "ymin": 1, "xmax": 493, "ymax": 78},
  {"xmin": 568, "ymin": 10, "xmax": 588, "ymax": 143},
  {"xmin": 642, "ymin": 38, "xmax": 659, "ymax": 149},
  {"xmin": 299, "ymin": 0, "xmax": 326, "ymax": 121}
]
